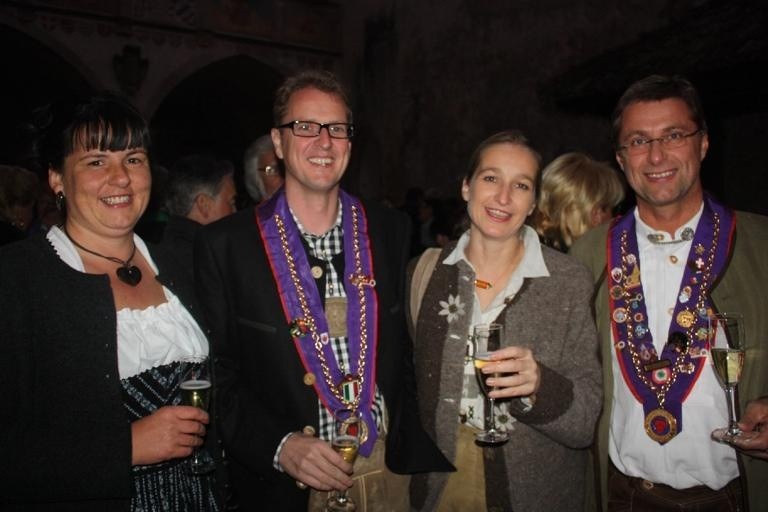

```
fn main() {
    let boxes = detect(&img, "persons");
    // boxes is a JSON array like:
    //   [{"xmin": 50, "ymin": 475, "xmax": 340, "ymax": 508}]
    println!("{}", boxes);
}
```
[
  {"xmin": 403, "ymin": 123, "xmax": 605, "ymax": 512},
  {"xmin": 563, "ymin": 67, "xmax": 768, "ymax": 511},
  {"xmin": 1, "ymin": 86, "xmax": 237, "ymax": 512},
  {"xmin": 190, "ymin": 67, "xmax": 459, "ymax": 511},
  {"xmin": 1, "ymin": 109, "xmax": 768, "ymax": 294}
]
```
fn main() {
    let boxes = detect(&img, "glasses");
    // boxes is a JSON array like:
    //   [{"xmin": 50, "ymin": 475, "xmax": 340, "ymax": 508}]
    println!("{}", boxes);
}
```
[
  {"xmin": 618, "ymin": 128, "xmax": 698, "ymax": 157},
  {"xmin": 259, "ymin": 165, "xmax": 283, "ymax": 176},
  {"xmin": 280, "ymin": 121, "xmax": 355, "ymax": 138}
]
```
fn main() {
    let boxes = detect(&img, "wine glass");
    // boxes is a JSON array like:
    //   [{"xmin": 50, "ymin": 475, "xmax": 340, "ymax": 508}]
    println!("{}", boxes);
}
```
[
  {"xmin": 178, "ymin": 355, "xmax": 214, "ymax": 474},
  {"xmin": 707, "ymin": 313, "xmax": 760, "ymax": 444},
  {"xmin": 331, "ymin": 410, "xmax": 364, "ymax": 512},
  {"xmin": 473, "ymin": 324, "xmax": 510, "ymax": 447}
]
